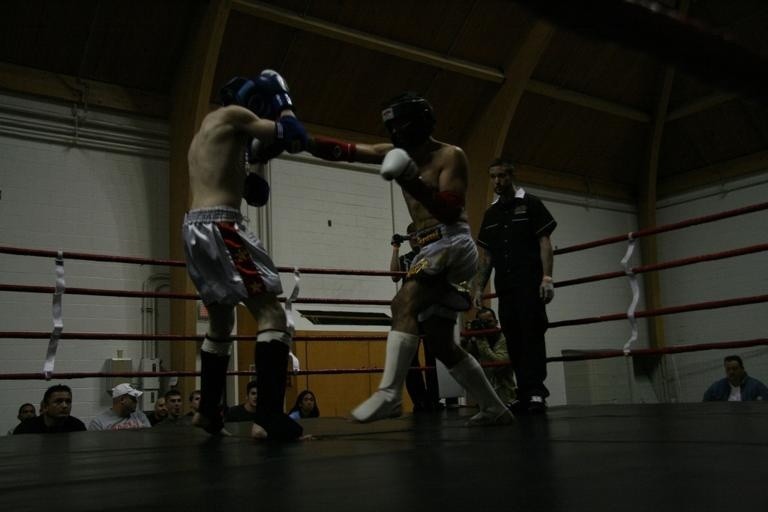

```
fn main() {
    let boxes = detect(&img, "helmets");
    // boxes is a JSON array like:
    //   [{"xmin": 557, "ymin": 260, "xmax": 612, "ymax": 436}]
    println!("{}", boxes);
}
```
[
  {"xmin": 381, "ymin": 95, "xmax": 435, "ymax": 145},
  {"xmin": 217, "ymin": 77, "xmax": 260, "ymax": 109}
]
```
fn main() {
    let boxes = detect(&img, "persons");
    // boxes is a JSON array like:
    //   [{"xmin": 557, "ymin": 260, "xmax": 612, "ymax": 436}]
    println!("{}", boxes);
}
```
[
  {"xmin": 290, "ymin": 388, "xmax": 322, "ymax": 421},
  {"xmin": 8, "ymin": 382, "xmax": 201, "ymax": 434},
  {"xmin": 702, "ymin": 354, "xmax": 768, "ymax": 401},
  {"xmin": 224, "ymin": 381, "xmax": 258, "ymax": 422},
  {"xmin": 390, "ymin": 222, "xmax": 443, "ymax": 411},
  {"xmin": 179, "ymin": 64, "xmax": 309, "ymax": 441},
  {"xmin": 305, "ymin": 87, "xmax": 519, "ymax": 425},
  {"xmin": 460, "ymin": 304, "xmax": 518, "ymax": 407},
  {"xmin": 470, "ymin": 157, "xmax": 558, "ymax": 415}
]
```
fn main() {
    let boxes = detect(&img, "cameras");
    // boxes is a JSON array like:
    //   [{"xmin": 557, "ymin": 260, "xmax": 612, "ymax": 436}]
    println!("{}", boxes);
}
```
[
  {"xmin": 391, "ymin": 232, "xmax": 410, "ymax": 245},
  {"xmin": 468, "ymin": 318, "xmax": 485, "ymax": 336}
]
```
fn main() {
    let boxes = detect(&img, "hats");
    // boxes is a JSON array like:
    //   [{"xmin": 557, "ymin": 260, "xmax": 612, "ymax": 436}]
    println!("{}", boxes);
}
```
[{"xmin": 108, "ymin": 381, "xmax": 144, "ymax": 398}]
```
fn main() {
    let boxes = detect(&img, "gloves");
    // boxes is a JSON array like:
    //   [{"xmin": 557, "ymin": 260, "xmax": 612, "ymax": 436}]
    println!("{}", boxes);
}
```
[
  {"xmin": 249, "ymin": 128, "xmax": 308, "ymax": 163},
  {"xmin": 539, "ymin": 276, "xmax": 556, "ymax": 304},
  {"xmin": 245, "ymin": 172, "xmax": 271, "ymax": 209},
  {"xmin": 257, "ymin": 69, "xmax": 299, "ymax": 114},
  {"xmin": 380, "ymin": 146, "xmax": 422, "ymax": 184}
]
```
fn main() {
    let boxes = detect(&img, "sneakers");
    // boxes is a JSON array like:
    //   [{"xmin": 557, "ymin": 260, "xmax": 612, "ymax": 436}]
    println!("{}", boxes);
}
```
[
  {"xmin": 507, "ymin": 397, "xmax": 528, "ymax": 412},
  {"xmin": 529, "ymin": 392, "xmax": 548, "ymax": 415}
]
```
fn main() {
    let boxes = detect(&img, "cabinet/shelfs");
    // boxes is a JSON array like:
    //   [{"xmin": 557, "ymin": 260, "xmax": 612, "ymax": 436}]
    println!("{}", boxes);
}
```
[{"xmin": 284, "ymin": 330, "xmax": 426, "ymax": 417}]
